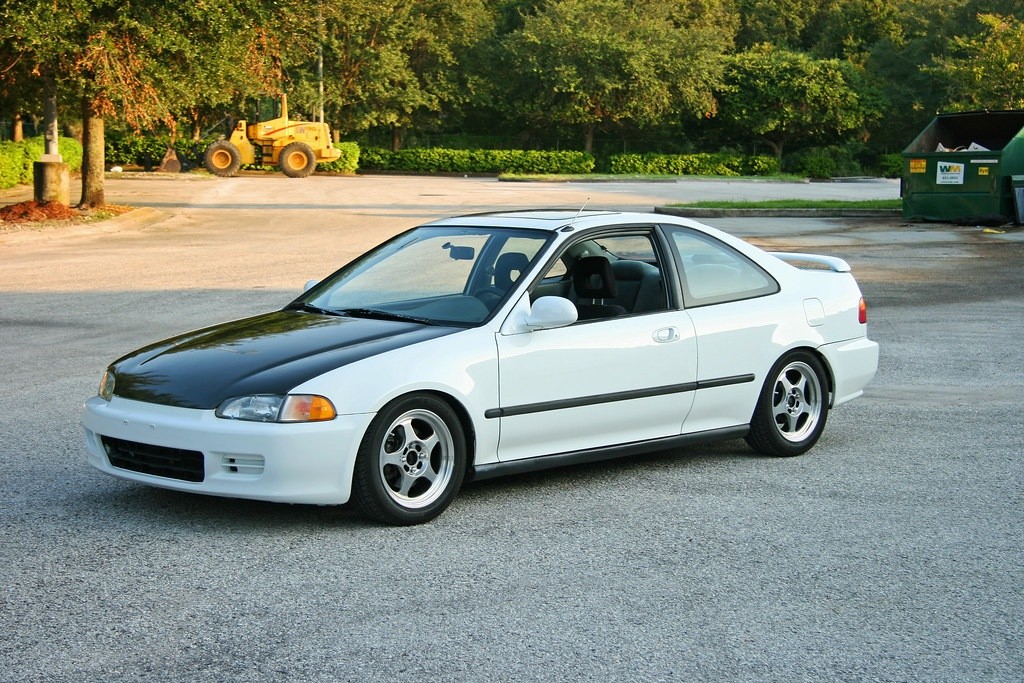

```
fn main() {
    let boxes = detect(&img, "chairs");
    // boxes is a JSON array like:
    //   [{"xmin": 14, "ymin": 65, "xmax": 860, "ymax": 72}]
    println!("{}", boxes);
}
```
[
  {"xmin": 485, "ymin": 253, "xmax": 534, "ymax": 313},
  {"xmin": 575, "ymin": 254, "xmax": 626, "ymax": 321}
]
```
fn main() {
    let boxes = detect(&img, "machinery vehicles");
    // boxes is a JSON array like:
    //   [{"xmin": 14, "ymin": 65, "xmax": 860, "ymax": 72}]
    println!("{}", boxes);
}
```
[{"xmin": 202, "ymin": 92, "xmax": 343, "ymax": 178}]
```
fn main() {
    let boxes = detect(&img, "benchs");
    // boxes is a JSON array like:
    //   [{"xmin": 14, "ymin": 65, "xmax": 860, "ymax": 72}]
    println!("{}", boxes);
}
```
[{"xmin": 568, "ymin": 260, "xmax": 742, "ymax": 314}]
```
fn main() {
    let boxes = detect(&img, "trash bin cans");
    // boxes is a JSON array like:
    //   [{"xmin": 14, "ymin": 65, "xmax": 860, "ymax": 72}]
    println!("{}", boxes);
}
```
[
  {"xmin": 1010, "ymin": 175, "xmax": 1024, "ymax": 224},
  {"xmin": 899, "ymin": 109, "xmax": 1024, "ymax": 224}
]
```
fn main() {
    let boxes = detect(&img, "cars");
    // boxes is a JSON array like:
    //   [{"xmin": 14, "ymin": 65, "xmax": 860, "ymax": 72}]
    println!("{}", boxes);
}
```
[{"xmin": 77, "ymin": 206, "xmax": 883, "ymax": 525}]
